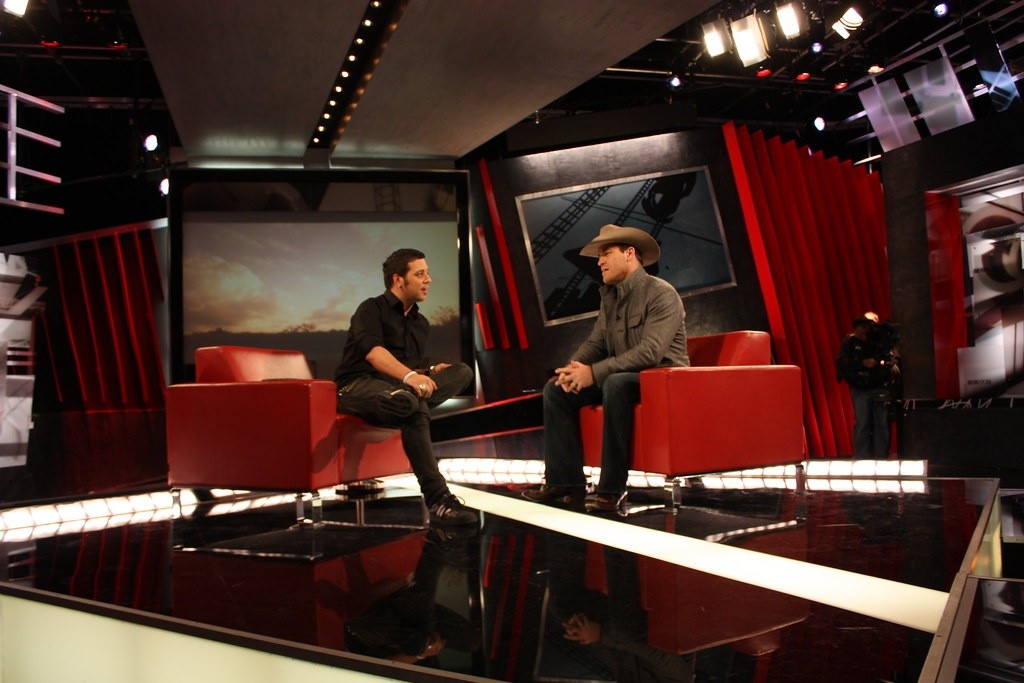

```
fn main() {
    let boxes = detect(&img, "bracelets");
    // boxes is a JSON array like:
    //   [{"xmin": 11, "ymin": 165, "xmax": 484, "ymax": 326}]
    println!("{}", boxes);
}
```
[
  {"xmin": 403, "ymin": 371, "xmax": 417, "ymax": 383},
  {"xmin": 424, "ymin": 366, "xmax": 434, "ymax": 376},
  {"xmin": 416, "ymin": 655, "xmax": 425, "ymax": 660}
]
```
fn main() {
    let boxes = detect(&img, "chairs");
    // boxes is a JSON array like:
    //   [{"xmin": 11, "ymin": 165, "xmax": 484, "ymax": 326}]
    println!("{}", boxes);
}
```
[{"xmin": 166, "ymin": 346, "xmax": 432, "ymax": 561}]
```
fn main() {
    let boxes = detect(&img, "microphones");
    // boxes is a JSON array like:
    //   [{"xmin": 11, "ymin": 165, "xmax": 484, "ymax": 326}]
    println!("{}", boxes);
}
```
[{"xmin": 615, "ymin": 315, "xmax": 621, "ymax": 321}]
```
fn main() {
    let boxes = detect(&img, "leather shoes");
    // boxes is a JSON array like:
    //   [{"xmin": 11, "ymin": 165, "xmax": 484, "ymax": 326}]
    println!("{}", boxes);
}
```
[
  {"xmin": 522, "ymin": 483, "xmax": 588, "ymax": 505},
  {"xmin": 583, "ymin": 491, "xmax": 629, "ymax": 511}
]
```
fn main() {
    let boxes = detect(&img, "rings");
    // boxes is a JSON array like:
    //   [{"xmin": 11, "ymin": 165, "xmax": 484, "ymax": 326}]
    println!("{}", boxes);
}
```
[
  {"xmin": 419, "ymin": 384, "xmax": 425, "ymax": 389},
  {"xmin": 428, "ymin": 645, "xmax": 432, "ymax": 648}
]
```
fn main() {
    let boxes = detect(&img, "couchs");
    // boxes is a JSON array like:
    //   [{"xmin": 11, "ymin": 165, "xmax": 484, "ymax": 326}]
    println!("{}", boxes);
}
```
[{"xmin": 548, "ymin": 330, "xmax": 810, "ymax": 546}]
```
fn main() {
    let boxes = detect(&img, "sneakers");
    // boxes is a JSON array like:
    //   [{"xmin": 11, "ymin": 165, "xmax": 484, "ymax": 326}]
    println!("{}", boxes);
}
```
[
  {"xmin": 375, "ymin": 387, "xmax": 419, "ymax": 419},
  {"xmin": 427, "ymin": 493, "xmax": 479, "ymax": 527}
]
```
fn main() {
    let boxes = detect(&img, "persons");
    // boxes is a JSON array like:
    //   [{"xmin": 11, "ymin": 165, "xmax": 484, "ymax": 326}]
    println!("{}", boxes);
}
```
[
  {"xmin": 837, "ymin": 312, "xmax": 905, "ymax": 459},
  {"xmin": 522, "ymin": 223, "xmax": 691, "ymax": 513},
  {"xmin": 344, "ymin": 512, "xmax": 649, "ymax": 683},
  {"xmin": 335, "ymin": 248, "xmax": 477, "ymax": 529}
]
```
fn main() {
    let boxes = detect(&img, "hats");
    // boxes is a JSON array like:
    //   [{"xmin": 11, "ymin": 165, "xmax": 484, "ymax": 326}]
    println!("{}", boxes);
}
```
[{"xmin": 578, "ymin": 223, "xmax": 661, "ymax": 267}]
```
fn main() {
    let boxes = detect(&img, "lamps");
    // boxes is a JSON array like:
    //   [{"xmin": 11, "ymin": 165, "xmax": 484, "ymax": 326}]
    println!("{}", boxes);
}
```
[{"xmin": 701, "ymin": 0, "xmax": 866, "ymax": 68}]
